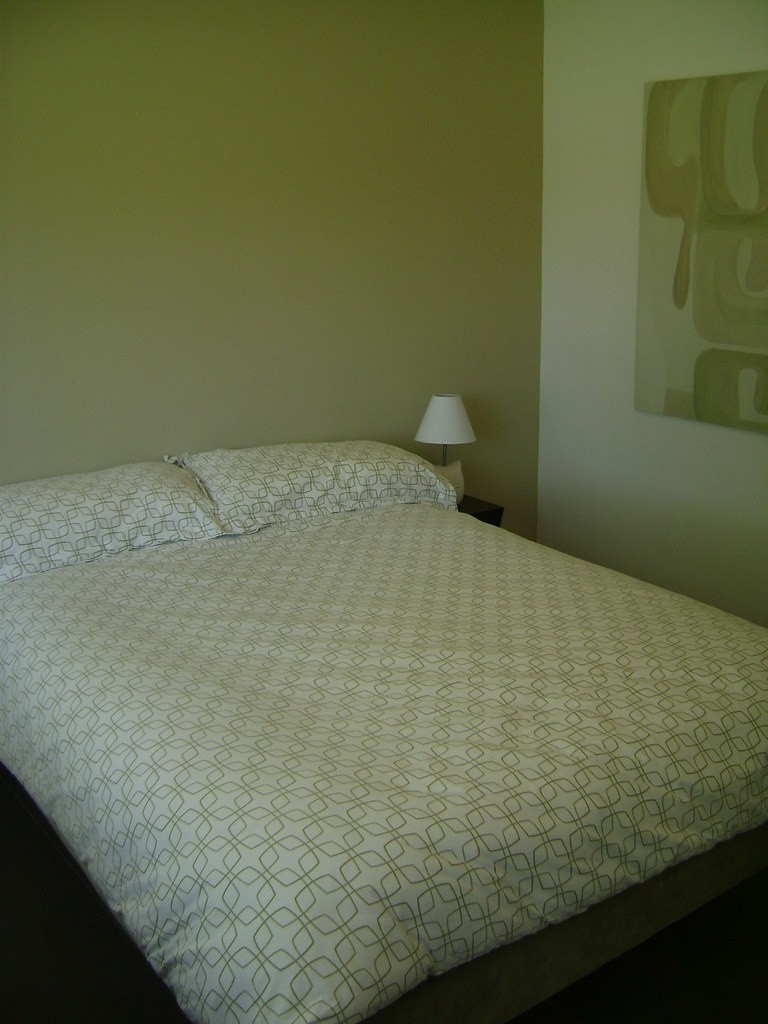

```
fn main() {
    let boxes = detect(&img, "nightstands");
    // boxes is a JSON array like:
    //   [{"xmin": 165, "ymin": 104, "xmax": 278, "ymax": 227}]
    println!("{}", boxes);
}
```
[{"xmin": 457, "ymin": 495, "xmax": 504, "ymax": 527}]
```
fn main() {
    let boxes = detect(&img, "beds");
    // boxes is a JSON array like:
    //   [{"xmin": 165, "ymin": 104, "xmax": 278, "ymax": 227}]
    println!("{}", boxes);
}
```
[{"xmin": 0, "ymin": 504, "xmax": 768, "ymax": 1024}]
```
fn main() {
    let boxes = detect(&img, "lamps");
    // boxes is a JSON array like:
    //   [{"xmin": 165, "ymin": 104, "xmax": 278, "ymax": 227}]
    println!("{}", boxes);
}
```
[{"xmin": 414, "ymin": 393, "xmax": 477, "ymax": 504}]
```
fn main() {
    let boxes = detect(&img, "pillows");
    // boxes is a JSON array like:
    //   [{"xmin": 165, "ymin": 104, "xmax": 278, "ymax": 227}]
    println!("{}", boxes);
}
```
[
  {"xmin": 164, "ymin": 440, "xmax": 458, "ymax": 536},
  {"xmin": 0, "ymin": 462, "xmax": 229, "ymax": 589}
]
